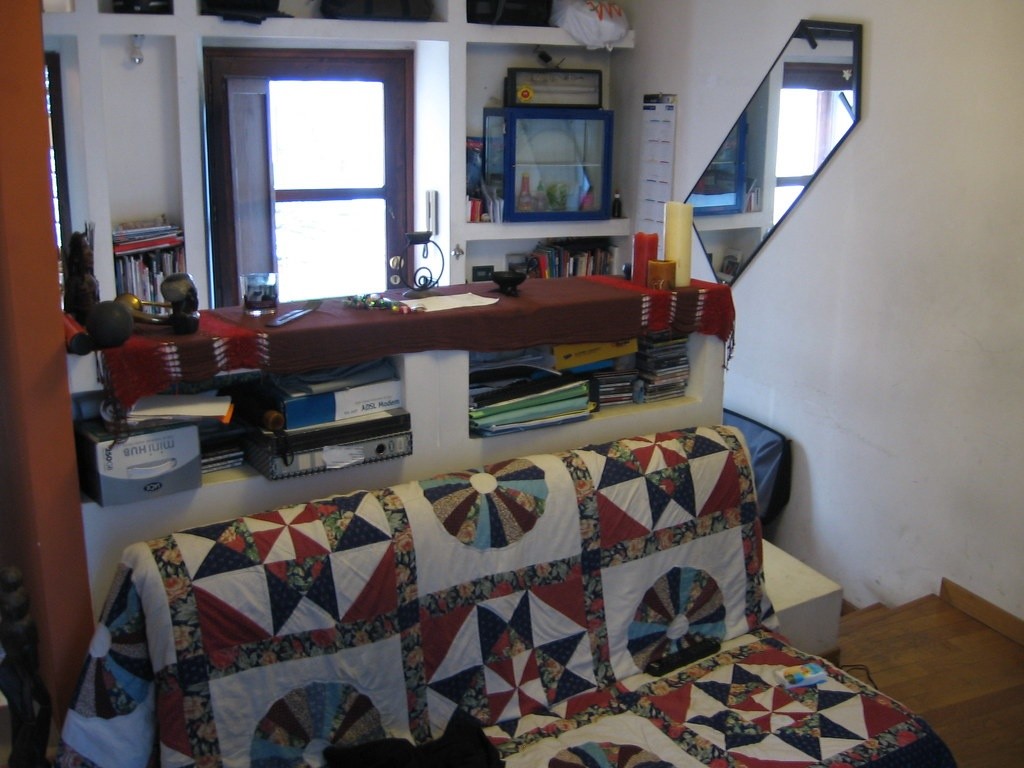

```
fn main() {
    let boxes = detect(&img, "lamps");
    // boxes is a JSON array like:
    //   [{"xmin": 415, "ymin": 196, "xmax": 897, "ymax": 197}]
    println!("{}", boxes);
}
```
[{"xmin": 130, "ymin": 33, "xmax": 144, "ymax": 65}]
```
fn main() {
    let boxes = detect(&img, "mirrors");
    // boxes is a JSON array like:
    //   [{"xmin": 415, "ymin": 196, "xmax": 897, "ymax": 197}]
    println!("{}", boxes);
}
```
[{"xmin": 683, "ymin": 18, "xmax": 864, "ymax": 288}]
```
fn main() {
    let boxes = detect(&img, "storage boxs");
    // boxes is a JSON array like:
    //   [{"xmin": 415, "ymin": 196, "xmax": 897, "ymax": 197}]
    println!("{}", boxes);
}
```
[
  {"xmin": 75, "ymin": 418, "xmax": 203, "ymax": 509},
  {"xmin": 551, "ymin": 338, "xmax": 638, "ymax": 371}
]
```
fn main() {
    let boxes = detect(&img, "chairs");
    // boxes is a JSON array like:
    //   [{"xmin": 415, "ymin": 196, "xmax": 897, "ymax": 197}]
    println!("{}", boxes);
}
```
[{"xmin": 722, "ymin": 409, "xmax": 794, "ymax": 544}]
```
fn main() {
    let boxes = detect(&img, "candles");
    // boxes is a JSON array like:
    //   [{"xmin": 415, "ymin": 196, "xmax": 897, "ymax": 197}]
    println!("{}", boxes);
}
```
[
  {"xmin": 663, "ymin": 201, "xmax": 692, "ymax": 288},
  {"xmin": 633, "ymin": 232, "xmax": 659, "ymax": 286},
  {"xmin": 647, "ymin": 259, "xmax": 676, "ymax": 290}
]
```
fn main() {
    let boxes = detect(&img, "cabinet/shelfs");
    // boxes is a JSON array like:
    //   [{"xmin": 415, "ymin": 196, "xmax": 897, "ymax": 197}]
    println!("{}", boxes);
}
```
[
  {"xmin": 41, "ymin": 0, "xmax": 635, "ymax": 309},
  {"xmin": 693, "ymin": 54, "xmax": 784, "ymax": 261}
]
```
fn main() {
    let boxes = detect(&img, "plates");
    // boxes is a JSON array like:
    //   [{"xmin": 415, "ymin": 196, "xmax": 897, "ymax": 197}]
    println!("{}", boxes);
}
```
[{"xmin": 490, "ymin": 270, "xmax": 526, "ymax": 295}]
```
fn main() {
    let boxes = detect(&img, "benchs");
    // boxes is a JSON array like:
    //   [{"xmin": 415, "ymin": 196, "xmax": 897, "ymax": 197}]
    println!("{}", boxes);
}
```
[{"xmin": 55, "ymin": 423, "xmax": 958, "ymax": 768}]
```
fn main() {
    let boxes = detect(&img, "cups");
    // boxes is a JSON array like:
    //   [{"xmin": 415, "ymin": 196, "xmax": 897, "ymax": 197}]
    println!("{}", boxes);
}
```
[
  {"xmin": 240, "ymin": 273, "xmax": 279, "ymax": 316},
  {"xmin": 535, "ymin": 179, "xmax": 547, "ymax": 211},
  {"xmin": 516, "ymin": 173, "xmax": 533, "ymax": 213}
]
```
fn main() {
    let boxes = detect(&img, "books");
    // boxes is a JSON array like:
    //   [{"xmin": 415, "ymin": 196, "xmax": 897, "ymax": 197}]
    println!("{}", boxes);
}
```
[
  {"xmin": 466, "ymin": 191, "xmax": 504, "ymax": 222},
  {"xmin": 128, "ymin": 332, "xmax": 691, "ymax": 480},
  {"xmin": 738, "ymin": 179, "xmax": 761, "ymax": 213},
  {"xmin": 531, "ymin": 241, "xmax": 619, "ymax": 278},
  {"xmin": 112, "ymin": 225, "xmax": 186, "ymax": 315}
]
